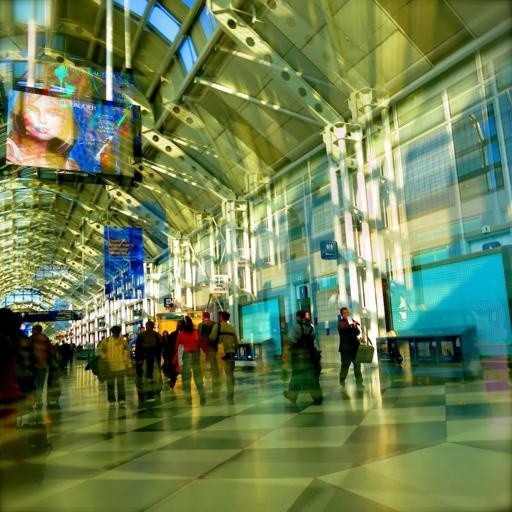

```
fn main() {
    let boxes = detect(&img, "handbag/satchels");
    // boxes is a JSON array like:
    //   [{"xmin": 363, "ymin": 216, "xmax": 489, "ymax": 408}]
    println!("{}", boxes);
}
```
[{"xmin": 355, "ymin": 344, "xmax": 374, "ymax": 363}]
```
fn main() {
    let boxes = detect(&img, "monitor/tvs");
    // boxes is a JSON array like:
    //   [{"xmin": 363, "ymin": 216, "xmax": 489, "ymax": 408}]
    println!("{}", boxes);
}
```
[{"xmin": 0, "ymin": 81, "xmax": 143, "ymax": 188}]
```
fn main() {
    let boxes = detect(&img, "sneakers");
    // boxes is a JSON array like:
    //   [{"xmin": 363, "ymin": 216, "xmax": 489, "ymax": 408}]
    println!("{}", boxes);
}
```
[
  {"xmin": 339, "ymin": 378, "xmax": 346, "ymax": 387},
  {"xmin": 107, "ymin": 392, "xmax": 235, "ymax": 405},
  {"xmin": 283, "ymin": 391, "xmax": 298, "ymax": 402},
  {"xmin": 357, "ymin": 384, "xmax": 365, "ymax": 389},
  {"xmin": 311, "ymin": 393, "xmax": 324, "ymax": 405}
]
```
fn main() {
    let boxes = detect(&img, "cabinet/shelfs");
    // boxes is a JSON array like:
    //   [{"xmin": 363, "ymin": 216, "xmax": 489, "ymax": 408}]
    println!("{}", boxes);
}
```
[{"xmin": 372, "ymin": 327, "xmax": 484, "ymax": 379}]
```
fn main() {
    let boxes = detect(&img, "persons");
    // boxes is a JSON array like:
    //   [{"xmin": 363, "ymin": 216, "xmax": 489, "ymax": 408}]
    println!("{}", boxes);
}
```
[
  {"xmin": 0, "ymin": 308, "xmax": 76, "ymax": 408},
  {"xmin": 338, "ymin": 307, "xmax": 363, "ymax": 387},
  {"xmin": 282, "ymin": 310, "xmax": 323, "ymax": 404},
  {"xmin": 6, "ymin": 91, "xmax": 81, "ymax": 171},
  {"xmin": 97, "ymin": 312, "xmax": 238, "ymax": 406}
]
